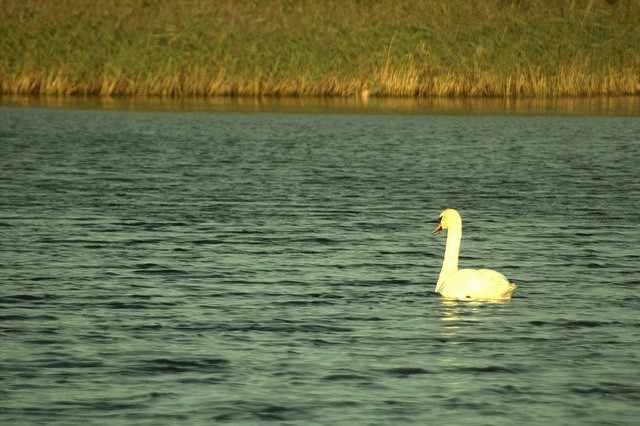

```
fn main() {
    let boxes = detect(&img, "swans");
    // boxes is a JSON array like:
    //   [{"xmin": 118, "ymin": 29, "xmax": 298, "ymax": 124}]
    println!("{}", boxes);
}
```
[{"xmin": 433, "ymin": 208, "xmax": 518, "ymax": 303}]
